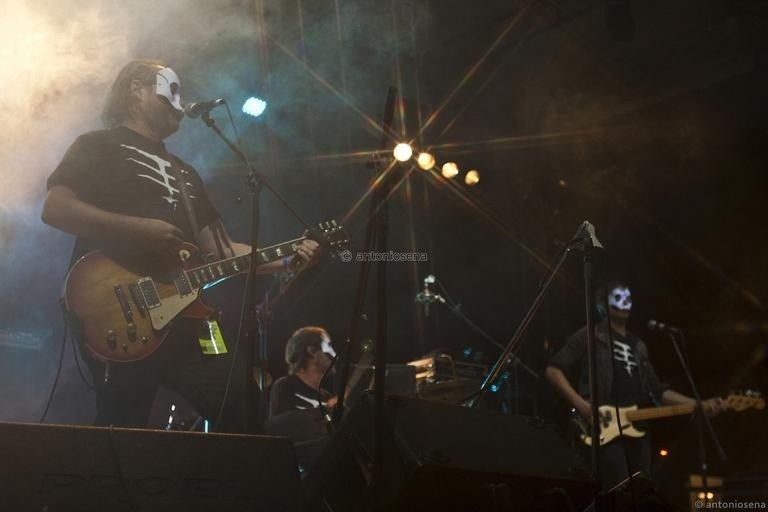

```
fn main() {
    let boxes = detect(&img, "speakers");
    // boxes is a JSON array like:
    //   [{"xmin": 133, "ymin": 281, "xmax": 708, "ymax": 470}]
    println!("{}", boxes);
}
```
[
  {"xmin": 304, "ymin": 392, "xmax": 593, "ymax": 511},
  {"xmin": 0, "ymin": 422, "xmax": 292, "ymax": 512}
]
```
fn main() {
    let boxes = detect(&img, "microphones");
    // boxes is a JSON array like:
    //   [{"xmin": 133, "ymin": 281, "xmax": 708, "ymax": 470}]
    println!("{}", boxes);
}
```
[
  {"xmin": 646, "ymin": 318, "xmax": 675, "ymax": 333},
  {"xmin": 422, "ymin": 284, "xmax": 434, "ymax": 318},
  {"xmin": 184, "ymin": 97, "xmax": 228, "ymax": 119}
]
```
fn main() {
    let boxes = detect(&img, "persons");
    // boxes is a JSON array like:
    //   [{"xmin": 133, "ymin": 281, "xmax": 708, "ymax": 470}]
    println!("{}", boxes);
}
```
[
  {"xmin": 545, "ymin": 280, "xmax": 727, "ymax": 493},
  {"xmin": 40, "ymin": 59, "xmax": 323, "ymax": 435},
  {"xmin": 266, "ymin": 327, "xmax": 340, "ymax": 479}
]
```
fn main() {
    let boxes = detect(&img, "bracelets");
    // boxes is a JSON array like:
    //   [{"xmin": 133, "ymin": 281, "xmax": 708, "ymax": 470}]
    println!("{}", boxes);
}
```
[{"xmin": 282, "ymin": 256, "xmax": 288, "ymax": 272}]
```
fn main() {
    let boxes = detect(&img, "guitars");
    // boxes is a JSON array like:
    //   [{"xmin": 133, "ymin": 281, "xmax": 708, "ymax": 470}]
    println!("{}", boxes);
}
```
[
  {"xmin": 61, "ymin": 221, "xmax": 351, "ymax": 366},
  {"xmin": 570, "ymin": 386, "xmax": 766, "ymax": 449}
]
